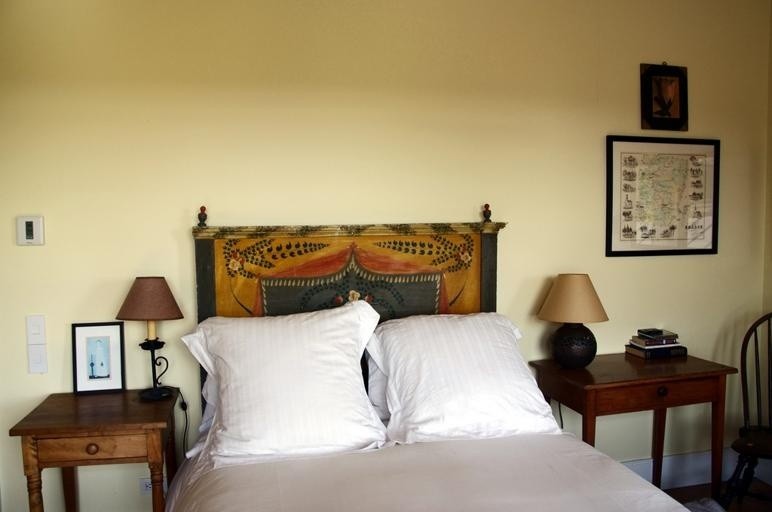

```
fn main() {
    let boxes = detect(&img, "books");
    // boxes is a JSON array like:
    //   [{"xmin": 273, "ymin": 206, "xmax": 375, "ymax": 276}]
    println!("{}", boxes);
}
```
[{"xmin": 624, "ymin": 328, "xmax": 687, "ymax": 359}]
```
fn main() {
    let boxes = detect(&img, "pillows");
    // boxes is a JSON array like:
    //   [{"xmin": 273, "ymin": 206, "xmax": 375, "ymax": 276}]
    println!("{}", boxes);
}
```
[
  {"xmin": 365, "ymin": 349, "xmax": 391, "ymax": 420},
  {"xmin": 367, "ymin": 311, "xmax": 564, "ymax": 445},
  {"xmin": 185, "ymin": 374, "xmax": 218, "ymax": 461},
  {"xmin": 181, "ymin": 299, "xmax": 396, "ymax": 464}
]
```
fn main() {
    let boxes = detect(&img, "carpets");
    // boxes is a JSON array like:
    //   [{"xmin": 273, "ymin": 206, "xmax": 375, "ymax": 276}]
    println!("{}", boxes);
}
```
[{"xmin": 684, "ymin": 498, "xmax": 725, "ymax": 511}]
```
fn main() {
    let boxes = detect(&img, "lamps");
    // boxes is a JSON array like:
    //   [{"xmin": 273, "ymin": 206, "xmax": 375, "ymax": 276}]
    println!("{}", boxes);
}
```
[
  {"xmin": 116, "ymin": 277, "xmax": 183, "ymax": 401},
  {"xmin": 536, "ymin": 273, "xmax": 609, "ymax": 371}
]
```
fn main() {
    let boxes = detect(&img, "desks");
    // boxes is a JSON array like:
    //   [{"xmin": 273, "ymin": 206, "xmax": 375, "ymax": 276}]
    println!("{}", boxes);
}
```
[
  {"xmin": 9, "ymin": 386, "xmax": 180, "ymax": 511},
  {"xmin": 528, "ymin": 353, "xmax": 738, "ymax": 504}
]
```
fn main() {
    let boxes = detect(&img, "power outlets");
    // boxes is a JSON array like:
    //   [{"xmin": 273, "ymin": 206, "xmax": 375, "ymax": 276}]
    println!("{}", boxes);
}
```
[{"xmin": 139, "ymin": 477, "xmax": 167, "ymax": 499}]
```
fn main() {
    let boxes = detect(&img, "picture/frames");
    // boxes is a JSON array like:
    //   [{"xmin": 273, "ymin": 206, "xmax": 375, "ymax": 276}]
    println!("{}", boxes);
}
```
[
  {"xmin": 605, "ymin": 135, "xmax": 720, "ymax": 256},
  {"xmin": 72, "ymin": 322, "xmax": 125, "ymax": 396},
  {"xmin": 640, "ymin": 63, "xmax": 689, "ymax": 131}
]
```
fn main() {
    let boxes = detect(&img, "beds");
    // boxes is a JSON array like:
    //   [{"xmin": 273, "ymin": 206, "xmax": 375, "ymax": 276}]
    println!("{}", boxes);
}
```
[{"xmin": 165, "ymin": 204, "xmax": 690, "ymax": 512}]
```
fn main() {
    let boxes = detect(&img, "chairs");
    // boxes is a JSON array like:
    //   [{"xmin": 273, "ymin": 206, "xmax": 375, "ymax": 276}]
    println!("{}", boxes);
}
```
[{"xmin": 721, "ymin": 313, "xmax": 772, "ymax": 511}]
